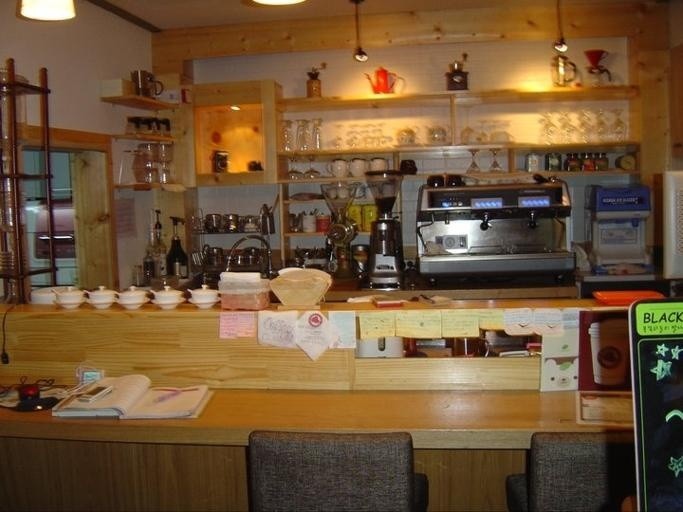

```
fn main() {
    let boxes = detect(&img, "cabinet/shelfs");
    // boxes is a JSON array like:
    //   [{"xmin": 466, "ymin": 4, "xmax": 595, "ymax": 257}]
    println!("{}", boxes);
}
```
[
  {"xmin": 0, "ymin": 58, "xmax": 58, "ymax": 303},
  {"xmin": 151, "ymin": 188, "xmax": 289, "ymax": 275},
  {"xmin": 100, "ymin": 94, "xmax": 184, "ymax": 192},
  {"xmin": 191, "ymin": 78, "xmax": 289, "ymax": 184},
  {"xmin": 276, "ymin": 84, "xmax": 642, "ymax": 267}
]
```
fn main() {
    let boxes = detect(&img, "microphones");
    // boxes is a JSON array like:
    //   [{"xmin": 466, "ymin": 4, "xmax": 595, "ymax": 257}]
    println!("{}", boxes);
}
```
[{"xmin": 1, "ymin": 303, "xmax": 19, "ymax": 365}]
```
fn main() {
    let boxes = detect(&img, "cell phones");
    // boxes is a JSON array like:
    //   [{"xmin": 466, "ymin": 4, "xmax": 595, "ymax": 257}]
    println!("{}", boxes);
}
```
[{"xmin": 78, "ymin": 384, "xmax": 113, "ymax": 403}]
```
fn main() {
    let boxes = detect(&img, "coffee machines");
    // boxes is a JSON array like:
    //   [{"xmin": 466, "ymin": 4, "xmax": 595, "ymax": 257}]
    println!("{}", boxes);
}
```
[{"xmin": 418, "ymin": 177, "xmax": 578, "ymax": 283}]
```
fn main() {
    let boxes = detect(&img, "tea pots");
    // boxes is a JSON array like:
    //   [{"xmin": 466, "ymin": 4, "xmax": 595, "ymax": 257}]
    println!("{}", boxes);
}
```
[{"xmin": 362, "ymin": 66, "xmax": 399, "ymax": 93}]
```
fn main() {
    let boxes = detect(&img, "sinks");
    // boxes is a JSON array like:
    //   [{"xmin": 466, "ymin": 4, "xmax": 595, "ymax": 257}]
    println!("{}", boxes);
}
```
[{"xmin": 181, "ymin": 269, "xmax": 222, "ymax": 303}]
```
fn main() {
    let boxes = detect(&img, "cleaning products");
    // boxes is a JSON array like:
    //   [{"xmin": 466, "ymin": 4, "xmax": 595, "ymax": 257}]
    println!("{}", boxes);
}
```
[{"xmin": 143, "ymin": 249, "xmax": 155, "ymax": 280}]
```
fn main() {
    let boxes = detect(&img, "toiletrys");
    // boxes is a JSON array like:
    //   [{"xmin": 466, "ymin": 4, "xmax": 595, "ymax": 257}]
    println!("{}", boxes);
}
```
[
  {"xmin": 166, "ymin": 216, "xmax": 190, "ymax": 279},
  {"xmin": 151, "ymin": 208, "xmax": 167, "ymax": 277}
]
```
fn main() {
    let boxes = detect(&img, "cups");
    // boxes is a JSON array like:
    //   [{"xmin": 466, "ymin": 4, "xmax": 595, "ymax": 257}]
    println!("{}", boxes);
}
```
[
  {"xmin": 547, "ymin": 53, "xmax": 577, "ymax": 88},
  {"xmin": 450, "ymin": 337, "xmax": 490, "ymax": 358},
  {"xmin": 130, "ymin": 69, "xmax": 163, "ymax": 100},
  {"xmin": 590, "ymin": 320, "xmax": 629, "ymax": 386},
  {"xmin": 125, "ymin": 116, "xmax": 172, "ymax": 136},
  {"xmin": 326, "ymin": 158, "xmax": 388, "ymax": 178},
  {"xmin": 582, "ymin": 66, "xmax": 613, "ymax": 85},
  {"xmin": 56, "ymin": 285, "xmax": 219, "ymax": 301},
  {"xmin": 288, "ymin": 212, "xmax": 333, "ymax": 232},
  {"xmin": 427, "ymin": 176, "xmax": 465, "ymax": 187},
  {"xmin": 584, "ymin": 47, "xmax": 609, "ymax": 66},
  {"xmin": 199, "ymin": 213, "xmax": 275, "ymax": 235},
  {"xmin": 393, "ymin": 127, "xmax": 450, "ymax": 146}
]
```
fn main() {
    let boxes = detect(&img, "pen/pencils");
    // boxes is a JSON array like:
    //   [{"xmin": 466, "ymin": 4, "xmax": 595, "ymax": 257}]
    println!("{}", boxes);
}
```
[{"xmin": 152, "ymin": 391, "xmax": 182, "ymax": 403}]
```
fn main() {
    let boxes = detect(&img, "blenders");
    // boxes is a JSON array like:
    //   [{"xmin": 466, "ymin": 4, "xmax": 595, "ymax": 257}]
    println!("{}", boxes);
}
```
[
  {"xmin": 320, "ymin": 184, "xmax": 357, "ymax": 280},
  {"xmin": 365, "ymin": 171, "xmax": 405, "ymax": 288}
]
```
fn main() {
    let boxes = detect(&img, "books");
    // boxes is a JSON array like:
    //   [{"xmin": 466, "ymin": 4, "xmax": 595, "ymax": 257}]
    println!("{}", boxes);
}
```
[{"xmin": 51, "ymin": 374, "xmax": 215, "ymax": 421}]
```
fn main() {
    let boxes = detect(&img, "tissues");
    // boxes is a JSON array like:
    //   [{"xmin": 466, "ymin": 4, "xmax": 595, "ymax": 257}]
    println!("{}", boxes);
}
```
[{"xmin": 218, "ymin": 271, "xmax": 272, "ymax": 311}]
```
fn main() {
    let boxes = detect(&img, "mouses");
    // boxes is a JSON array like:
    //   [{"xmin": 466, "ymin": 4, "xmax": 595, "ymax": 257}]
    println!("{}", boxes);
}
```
[{"xmin": 19, "ymin": 383, "xmax": 40, "ymax": 402}]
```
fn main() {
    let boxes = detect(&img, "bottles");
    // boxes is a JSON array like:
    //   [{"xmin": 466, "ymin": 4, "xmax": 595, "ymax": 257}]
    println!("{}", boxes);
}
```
[
  {"xmin": 208, "ymin": 247, "xmax": 258, "ymax": 265},
  {"xmin": 349, "ymin": 202, "xmax": 377, "ymax": 231},
  {"xmin": 306, "ymin": 69, "xmax": 322, "ymax": 96},
  {"xmin": 130, "ymin": 221, "xmax": 187, "ymax": 285},
  {"xmin": 209, "ymin": 148, "xmax": 229, "ymax": 175},
  {"xmin": 279, "ymin": 117, "xmax": 325, "ymax": 152},
  {"xmin": 565, "ymin": 151, "xmax": 610, "ymax": 170},
  {"xmin": 400, "ymin": 160, "xmax": 416, "ymax": 174}
]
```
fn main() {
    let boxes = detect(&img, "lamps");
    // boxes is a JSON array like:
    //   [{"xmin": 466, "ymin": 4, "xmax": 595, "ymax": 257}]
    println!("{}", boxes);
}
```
[
  {"xmin": 351, "ymin": 0, "xmax": 368, "ymax": 62},
  {"xmin": 16, "ymin": 0, "xmax": 76, "ymax": 21},
  {"xmin": 554, "ymin": 0, "xmax": 568, "ymax": 52}
]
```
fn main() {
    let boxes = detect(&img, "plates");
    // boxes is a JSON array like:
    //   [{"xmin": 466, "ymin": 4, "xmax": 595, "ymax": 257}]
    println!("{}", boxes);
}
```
[{"xmin": 47, "ymin": 302, "xmax": 220, "ymax": 311}]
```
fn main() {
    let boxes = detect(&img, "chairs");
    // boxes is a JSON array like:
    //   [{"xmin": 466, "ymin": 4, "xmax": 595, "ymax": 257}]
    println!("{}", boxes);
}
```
[
  {"xmin": 505, "ymin": 431, "xmax": 638, "ymax": 512},
  {"xmin": 249, "ymin": 430, "xmax": 428, "ymax": 512}
]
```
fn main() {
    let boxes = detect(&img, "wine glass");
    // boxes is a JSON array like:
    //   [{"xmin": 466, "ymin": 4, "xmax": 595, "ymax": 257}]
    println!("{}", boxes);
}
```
[
  {"xmin": 467, "ymin": 149, "xmax": 502, "ymax": 171},
  {"xmin": 332, "ymin": 122, "xmax": 391, "ymax": 149},
  {"xmin": 539, "ymin": 108, "xmax": 626, "ymax": 143},
  {"xmin": 139, "ymin": 143, "xmax": 172, "ymax": 184},
  {"xmin": 285, "ymin": 154, "xmax": 321, "ymax": 179},
  {"xmin": 456, "ymin": 119, "xmax": 513, "ymax": 144}
]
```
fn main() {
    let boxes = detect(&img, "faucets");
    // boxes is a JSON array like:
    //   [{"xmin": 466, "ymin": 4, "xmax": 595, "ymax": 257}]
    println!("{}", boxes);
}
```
[
  {"xmin": 225, "ymin": 234, "xmax": 273, "ymax": 273},
  {"xmin": 201, "ymin": 243, "xmax": 210, "ymax": 284}
]
